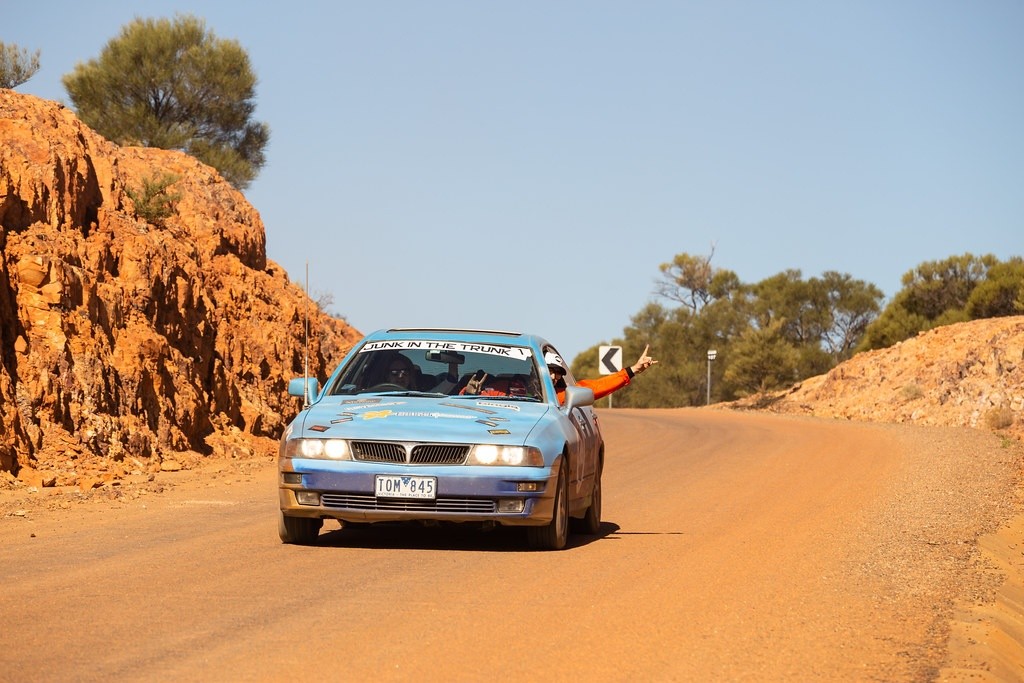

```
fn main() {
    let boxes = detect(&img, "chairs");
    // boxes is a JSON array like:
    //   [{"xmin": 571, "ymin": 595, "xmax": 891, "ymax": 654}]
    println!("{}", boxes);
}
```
[{"xmin": 366, "ymin": 373, "xmax": 438, "ymax": 393}]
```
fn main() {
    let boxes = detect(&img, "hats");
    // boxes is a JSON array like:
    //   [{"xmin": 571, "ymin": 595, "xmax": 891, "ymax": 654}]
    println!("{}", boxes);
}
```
[{"xmin": 530, "ymin": 352, "xmax": 567, "ymax": 376}]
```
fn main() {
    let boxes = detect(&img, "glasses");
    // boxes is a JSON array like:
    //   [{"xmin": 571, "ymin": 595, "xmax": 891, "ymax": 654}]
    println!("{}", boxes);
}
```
[{"xmin": 390, "ymin": 368, "xmax": 409, "ymax": 376}]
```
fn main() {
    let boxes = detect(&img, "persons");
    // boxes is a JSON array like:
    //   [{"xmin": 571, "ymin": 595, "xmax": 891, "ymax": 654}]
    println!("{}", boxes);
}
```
[
  {"xmin": 458, "ymin": 343, "xmax": 659, "ymax": 407},
  {"xmin": 383, "ymin": 349, "xmax": 460, "ymax": 396}
]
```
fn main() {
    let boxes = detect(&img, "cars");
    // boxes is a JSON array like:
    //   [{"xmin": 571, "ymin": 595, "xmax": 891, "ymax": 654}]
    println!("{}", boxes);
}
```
[{"xmin": 274, "ymin": 328, "xmax": 606, "ymax": 551}]
